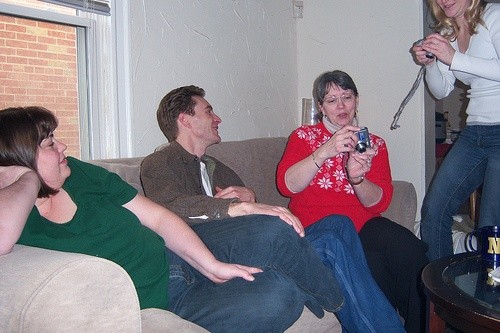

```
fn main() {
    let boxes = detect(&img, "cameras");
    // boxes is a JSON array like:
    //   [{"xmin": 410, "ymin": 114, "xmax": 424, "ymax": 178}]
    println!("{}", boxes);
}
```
[
  {"xmin": 344, "ymin": 127, "xmax": 370, "ymax": 153},
  {"xmin": 415, "ymin": 39, "xmax": 435, "ymax": 59}
]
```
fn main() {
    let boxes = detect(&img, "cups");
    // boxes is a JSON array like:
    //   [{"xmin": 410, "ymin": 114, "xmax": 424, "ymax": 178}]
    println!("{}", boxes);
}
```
[{"xmin": 470, "ymin": 226, "xmax": 500, "ymax": 259}]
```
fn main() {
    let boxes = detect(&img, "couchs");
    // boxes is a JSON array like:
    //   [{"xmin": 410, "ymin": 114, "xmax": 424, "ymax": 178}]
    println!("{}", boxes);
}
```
[{"xmin": 0, "ymin": 136, "xmax": 418, "ymax": 333}]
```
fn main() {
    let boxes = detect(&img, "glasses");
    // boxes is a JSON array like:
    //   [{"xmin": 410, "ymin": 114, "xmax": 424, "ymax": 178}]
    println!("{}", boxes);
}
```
[{"xmin": 321, "ymin": 91, "xmax": 356, "ymax": 105}]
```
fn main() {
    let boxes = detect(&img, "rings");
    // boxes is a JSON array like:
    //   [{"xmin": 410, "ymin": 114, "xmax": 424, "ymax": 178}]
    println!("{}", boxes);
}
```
[
  {"xmin": 368, "ymin": 157, "xmax": 371, "ymax": 162},
  {"xmin": 368, "ymin": 162, "xmax": 370, "ymax": 167}
]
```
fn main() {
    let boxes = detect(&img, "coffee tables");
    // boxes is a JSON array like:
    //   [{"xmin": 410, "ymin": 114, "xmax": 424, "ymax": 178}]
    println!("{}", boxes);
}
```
[{"xmin": 421, "ymin": 248, "xmax": 500, "ymax": 333}]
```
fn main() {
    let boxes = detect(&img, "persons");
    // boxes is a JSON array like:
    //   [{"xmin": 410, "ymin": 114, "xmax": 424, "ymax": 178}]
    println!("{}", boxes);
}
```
[
  {"xmin": 139, "ymin": 85, "xmax": 404, "ymax": 333},
  {"xmin": 276, "ymin": 70, "xmax": 430, "ymax": 333},
  {"xmin": 0, "ymin": 105, "xmax": 347, "ymax": 333},
  {"xmin": 412, "ymin": 0, "xmax": 500, "ymax": 261}
]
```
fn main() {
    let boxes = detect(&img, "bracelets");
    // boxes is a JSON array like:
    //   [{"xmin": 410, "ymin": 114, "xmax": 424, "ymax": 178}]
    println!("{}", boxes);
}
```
[
  {"xmin": 312, "ymin": 152, "xmax": 321, "ymax": 169},
  {"xmin": 349, "ymin": 175, "xmax": 365, "ymax": 185}
]
